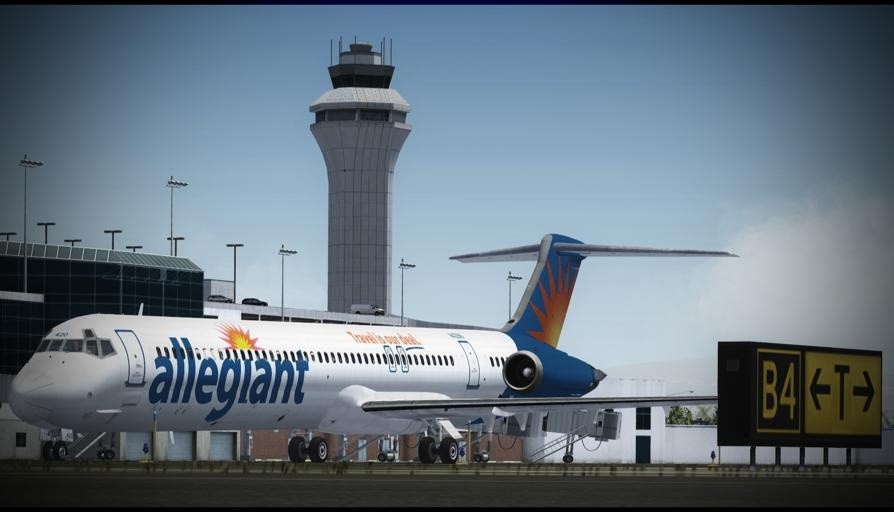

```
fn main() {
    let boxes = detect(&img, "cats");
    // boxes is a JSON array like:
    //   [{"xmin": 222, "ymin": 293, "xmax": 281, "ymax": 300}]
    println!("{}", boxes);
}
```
[{"xmin": 8, "ymin": 232, "xmax": 739, "ymax": 463}]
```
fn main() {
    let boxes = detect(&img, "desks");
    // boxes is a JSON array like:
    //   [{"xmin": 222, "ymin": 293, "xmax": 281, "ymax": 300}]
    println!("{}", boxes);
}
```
[
  {"xmin": 227, "ymin": 243, "xmax": 244, "ymax": 304},
  {"xmin": 2, "ymin": 156, "xmax": 186, "ymax": 292},
  {"xmin": 509, "ymin": 272, "xmax": 521, "ymax": 320},
  {"xmin": 398, "ymin": 259, "xmax": 415, "ymax": 327},
  {"xmin": 278, "ymin": 245, "xmax": 298, "ymax": 320}
]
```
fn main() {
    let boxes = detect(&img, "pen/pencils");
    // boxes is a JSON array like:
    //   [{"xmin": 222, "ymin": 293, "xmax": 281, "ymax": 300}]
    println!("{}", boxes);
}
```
[
  {"xmin": 207, "ymin": 294, "xmax": 231, "ymax": 303},
  {"xmin": 242, "ymin": 297, "xmax": 267, "ymax": 306}
]
